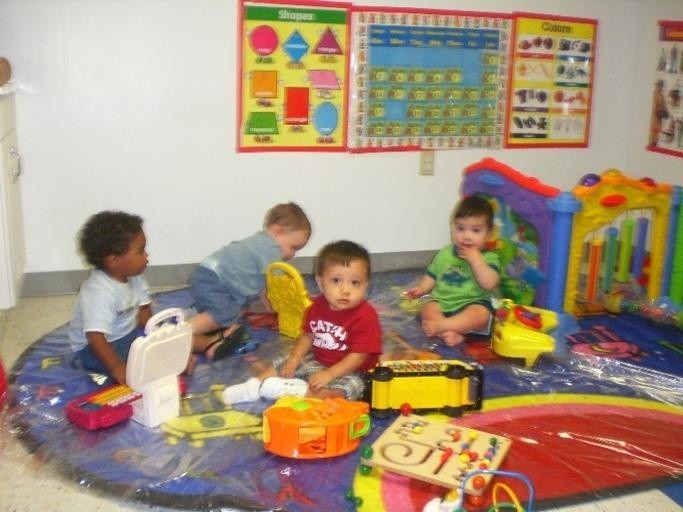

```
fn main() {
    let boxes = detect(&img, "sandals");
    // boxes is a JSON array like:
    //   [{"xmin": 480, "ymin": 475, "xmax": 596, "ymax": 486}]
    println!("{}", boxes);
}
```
[{"xmin": 204, "ymin": 325, "xmax": 243, "ymax": 361}]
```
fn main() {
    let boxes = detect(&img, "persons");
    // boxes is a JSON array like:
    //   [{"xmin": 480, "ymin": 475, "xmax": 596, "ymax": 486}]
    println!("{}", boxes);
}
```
[
  {"xmin": 63, "ymin": 211, "xmax": 246, "ymax": 381},
  {"xmin": 218, "ymin": 242, "xmax": 382, "ymax": 404},
  {"xmin": 401, "ymin": 199, "xmax": 502, "ymax": 348},
  {"xmin": 177, "ymin": 203, "xmax": 310, "ymax": 344}
]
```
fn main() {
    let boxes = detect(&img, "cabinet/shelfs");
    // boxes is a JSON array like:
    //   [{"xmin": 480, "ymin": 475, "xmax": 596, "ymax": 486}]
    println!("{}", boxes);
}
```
[{"xmin": 0, "ymin": 85, "xmax": 25, "ymax": 312}]
